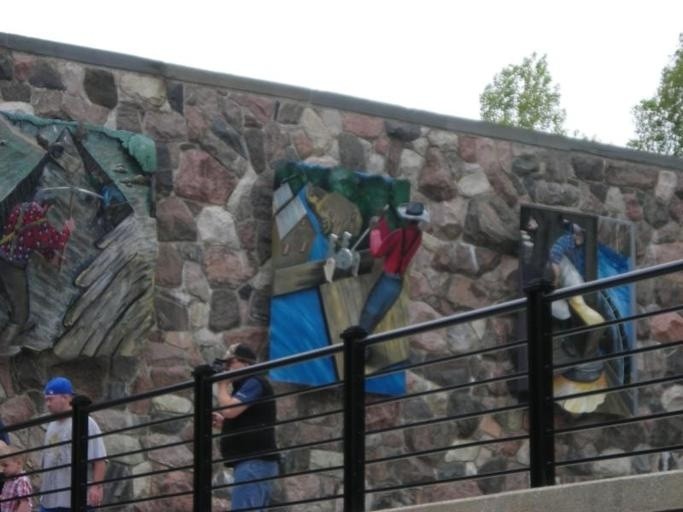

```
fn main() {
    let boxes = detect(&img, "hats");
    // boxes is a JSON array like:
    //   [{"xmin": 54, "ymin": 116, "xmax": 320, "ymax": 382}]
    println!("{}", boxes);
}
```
[
  {"xmin": 43, "ymin": 377, "xmax": 77, "ymax": 396},
  {"xmin": 216, "ymin": 343, "xmax": 256, "ymax": 364},
  {"xmin": 396, "ymin": 203, "xmax": 431, "ymax": 223}
]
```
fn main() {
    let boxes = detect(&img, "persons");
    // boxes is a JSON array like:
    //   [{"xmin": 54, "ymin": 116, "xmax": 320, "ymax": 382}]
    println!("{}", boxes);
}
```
[
  {"xmin": 211, "ymin": 341, "xmax": 281, "ymax": 511},
  {"xmin": 0, "ymin": 418, "xmax": 11, "ymax": 492},
  {"xmin": 357, "ymin": 200, "xmax": 430, "ymax": 361},
  {"xmin": 544, "ymin": 230, "xmax": 585, "ymax": 357},
  {"xmin": 0, "ymin": 190, "xmax": 75, "ymax": 354},
  {"xmin": 39, "ymin": 376, "xmax": 108, "ymax": 512},
  {"xmin": 0, "ymin": 445, "xmax": 33, "ymax": 512}
]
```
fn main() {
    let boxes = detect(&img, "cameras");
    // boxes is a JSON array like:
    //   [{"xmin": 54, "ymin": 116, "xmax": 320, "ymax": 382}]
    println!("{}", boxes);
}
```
[{"xmin": 213, "ymin": 358, "xmax": 230, "ymax": 376}]
